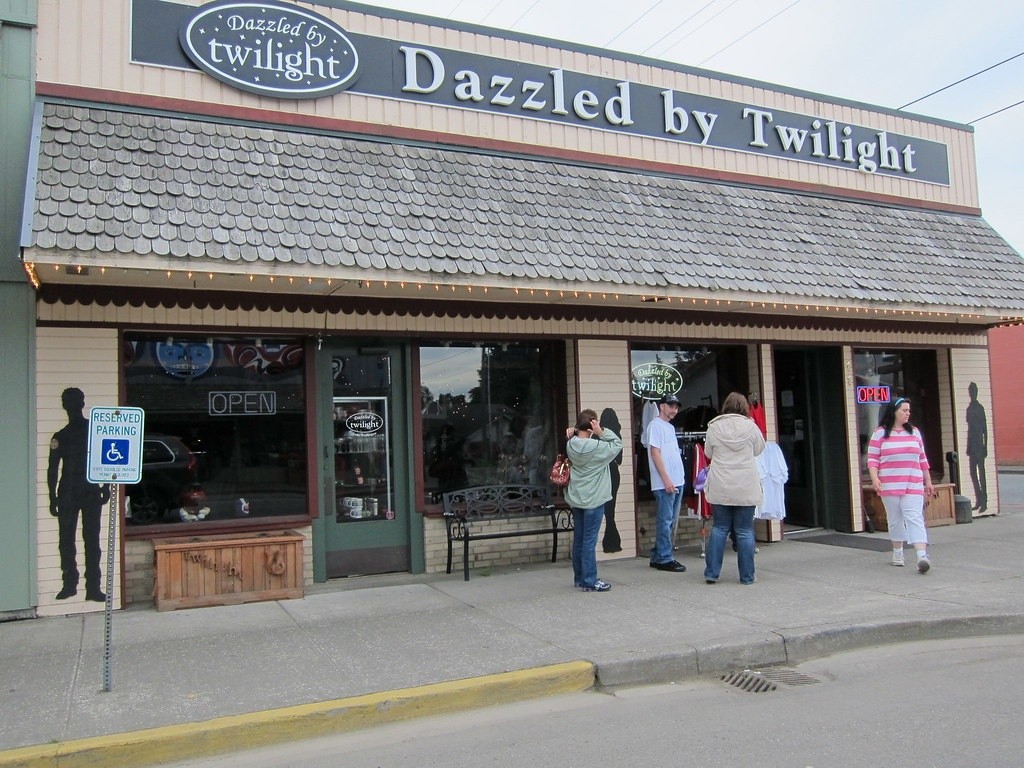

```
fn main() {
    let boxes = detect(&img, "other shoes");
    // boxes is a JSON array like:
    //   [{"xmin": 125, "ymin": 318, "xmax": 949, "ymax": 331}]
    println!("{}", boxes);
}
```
[
  {"xmin": 740, "ymin": 574, "xmax": 757, "ymax": 586},
  {"xmin": 892, "ymin": 551, "xmax": 905, "ymax": 566},
  {"xmin": 915, "ymin": 554, "xmax": 932, "ymax": 572},
  {"xmin": 704, "ymin": 576, "xmax": 721, "ymax": 584},
  {"xmin": 656, "ymin": 560, "xmax": 686, "ymax": 572},
  {"xmin": 649, "ymin": 562, "xmax": 659, "ymax": 567},
  {"xmin": 574, "ymin": 577, "xmax": 602, "ymax": 588},
  {"xmin": 582, "ymin": 580, "xmax": 611, "ymax": 592}
]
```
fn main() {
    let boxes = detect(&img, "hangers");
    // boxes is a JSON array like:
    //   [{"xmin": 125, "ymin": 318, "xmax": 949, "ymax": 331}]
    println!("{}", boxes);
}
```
[{"xmin": 678, "ymin": 431, "xmax": 707, "ymax": 447}]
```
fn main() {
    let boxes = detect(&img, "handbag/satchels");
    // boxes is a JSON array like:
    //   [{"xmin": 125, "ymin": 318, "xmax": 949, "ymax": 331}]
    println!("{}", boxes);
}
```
[
  {"xmin": 692, "ymin": 466, "xmax": 710, "ymax": 491},
  {"xmin": 548, "ymin": 436, "xmax": 573, "ymax": 488}
]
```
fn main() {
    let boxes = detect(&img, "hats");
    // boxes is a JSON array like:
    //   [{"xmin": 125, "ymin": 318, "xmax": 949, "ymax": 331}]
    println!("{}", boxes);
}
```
[{"xmin": 659, "ymin": 392, "xmax": 682, "ymax": 407}]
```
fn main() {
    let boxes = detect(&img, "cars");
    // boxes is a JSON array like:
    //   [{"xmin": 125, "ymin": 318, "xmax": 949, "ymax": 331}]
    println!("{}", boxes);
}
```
[{"xmin": 126, "ymin": 434, "xmax": 208, "ymax": 526}]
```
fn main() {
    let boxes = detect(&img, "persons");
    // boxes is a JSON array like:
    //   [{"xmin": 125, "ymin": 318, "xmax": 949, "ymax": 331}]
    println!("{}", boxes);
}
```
[
  {"xmin": 646, "ymin": 395, "xmax": 687, "ymax": 572},
  {"xmin": 562, "ymin": 408, "xmax": 624, "ymax": 593},
  {"xmin": 703, "ymin": 391, "xmax": 766, "ymax": 585},
  {"xmin": 867, "ymin": 397, "xmax": 934, "ymax": 572},
  {"xmin": 429, "ymin": 424, "xmax": 471, "ymax": 493},
  {"xmin": 497, "ymin": 416, "xmax": 546, "ymax": 499}
]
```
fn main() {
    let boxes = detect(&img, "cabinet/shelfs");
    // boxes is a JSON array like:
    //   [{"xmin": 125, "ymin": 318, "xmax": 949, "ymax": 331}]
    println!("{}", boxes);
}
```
[{"xmin": 333, "ymin": 396, "xmax": 390, "ymax": 524}]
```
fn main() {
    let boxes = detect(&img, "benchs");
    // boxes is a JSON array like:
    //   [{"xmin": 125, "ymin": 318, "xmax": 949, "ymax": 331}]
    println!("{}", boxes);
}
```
[{"xmin": 442, "ymin": 485, "xmax": 574, "ymax": 583}]
